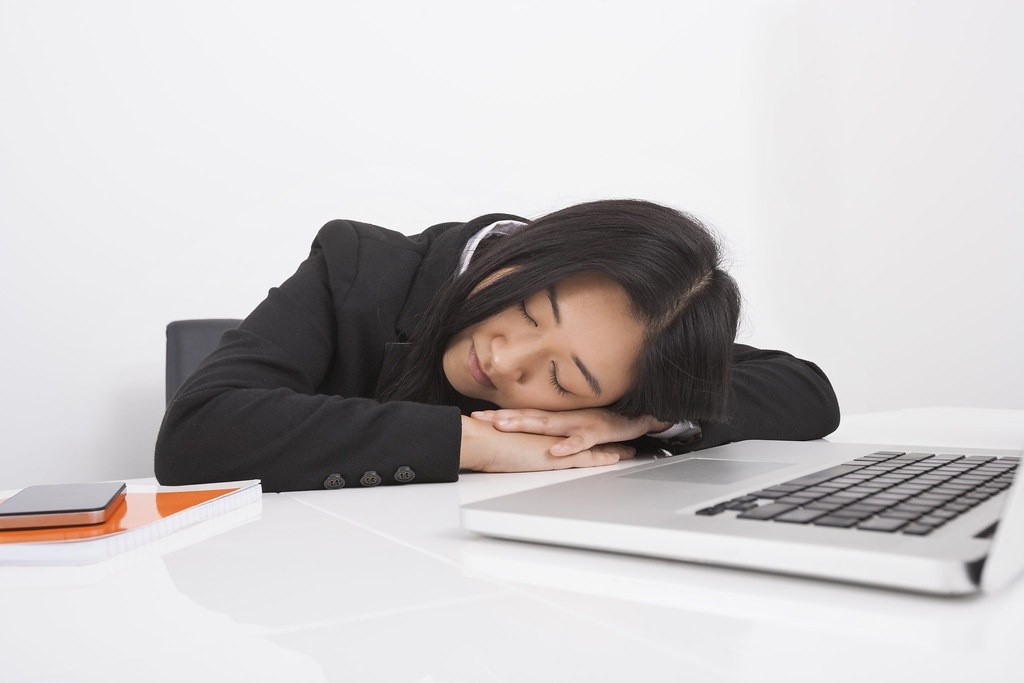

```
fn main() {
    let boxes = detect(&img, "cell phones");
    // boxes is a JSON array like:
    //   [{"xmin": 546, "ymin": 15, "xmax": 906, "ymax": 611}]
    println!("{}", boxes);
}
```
[{"xmin": 0, "ymin": 482, "xmax": 127, "ymax": 529}]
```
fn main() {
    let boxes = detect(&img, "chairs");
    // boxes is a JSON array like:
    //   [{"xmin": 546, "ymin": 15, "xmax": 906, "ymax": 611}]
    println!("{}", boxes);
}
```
[{"xmin": 164, "ymin": 319, "xmax": 243, "ymax": 414}]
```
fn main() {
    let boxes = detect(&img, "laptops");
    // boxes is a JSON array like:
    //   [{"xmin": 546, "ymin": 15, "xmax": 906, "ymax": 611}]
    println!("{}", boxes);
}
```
[{"xmin": 457, "ymin": 439, "xmax": 1024, "ymax": 594}]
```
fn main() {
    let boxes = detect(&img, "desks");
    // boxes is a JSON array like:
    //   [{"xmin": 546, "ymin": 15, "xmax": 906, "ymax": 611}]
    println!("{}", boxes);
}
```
[{"xmin": 0, "ymin": 400, "xmax": 1023, "ymax": 682}]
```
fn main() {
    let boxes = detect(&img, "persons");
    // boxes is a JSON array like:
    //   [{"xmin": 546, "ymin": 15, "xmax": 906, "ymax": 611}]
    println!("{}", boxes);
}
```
[{"xmin": 151, "ymin": 197, "xmax": 843, "ymax": 494}]
